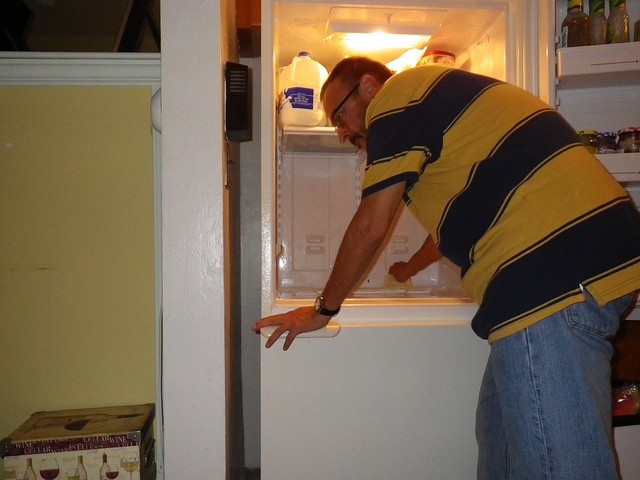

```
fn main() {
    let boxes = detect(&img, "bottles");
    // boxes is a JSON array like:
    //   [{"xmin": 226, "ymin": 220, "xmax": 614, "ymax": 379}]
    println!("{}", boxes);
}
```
[
  {"xmin": 34, "ymin": 412, "xmax": 144, "ymax": 429},
  {"xmin": 99, "ymin": 453, "xmax": 112, "ymax": 480},
  {"xmin": 608, "ymin": 0, "xmax": 630, "ymax": 45},
  {"xmin": 75, "ymin": 456, "xmax": 87, "ymax": 480},
  {"xmin": 561, "ymin": 0, "xmax": 590, "ymax": 48},
  {"xmin": 588, "ymin": 0, "xmax": 608, "ymax": 45},
  {"xmin": 23, "ymin": 457, "xmax": 36, "ymax": 480}
]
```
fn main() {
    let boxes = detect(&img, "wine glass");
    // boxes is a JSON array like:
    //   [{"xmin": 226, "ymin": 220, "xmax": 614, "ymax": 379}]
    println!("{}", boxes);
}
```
[
  {"xmin": 66, "ymin": 471, "xmax": 80, "ymax": 480},
  {"xmin": 119, "ymin": 451, "xmax": 139, "ymax": 480},
  {"xmin": 105, "ymin": 464, "xmax": 119, "ymax": 480},
  {"xmin": 39, "ymin": 458, "xmax": 60, "ymax": 480},
  {"xmin": 24, "ymin": 419, "xmax": 92, "ymax": 434}
]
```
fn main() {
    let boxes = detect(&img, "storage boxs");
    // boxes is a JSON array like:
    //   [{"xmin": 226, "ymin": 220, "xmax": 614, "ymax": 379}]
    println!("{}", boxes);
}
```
[{"xmin": 1, "ymin": 402, "xmax": 156, "ymax": 479}]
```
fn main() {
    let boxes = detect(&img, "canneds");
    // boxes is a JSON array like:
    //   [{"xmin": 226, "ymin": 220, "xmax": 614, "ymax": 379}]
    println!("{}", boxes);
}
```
[
  {"xmin": 616, "ymin": 127, "xmax": 640, "ymax": 153},
  {"xmin": 596, "ymin": 132, "xmax": 616, "ymax": 155},
  {"xmin": 575, "ymin": 130, "xmax": 598, "ymax": 156}
]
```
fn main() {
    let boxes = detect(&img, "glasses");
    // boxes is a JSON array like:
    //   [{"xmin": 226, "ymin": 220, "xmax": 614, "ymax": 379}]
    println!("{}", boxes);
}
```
[{"xmin": 331, "ymin": 83, "xmax": 359, "ymax": 126}]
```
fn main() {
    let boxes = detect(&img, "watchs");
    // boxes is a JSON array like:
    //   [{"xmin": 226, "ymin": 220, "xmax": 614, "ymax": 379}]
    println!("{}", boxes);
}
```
[{"xmin": 314, "ymin": 296, "xmax": 342, "ymax": 316}]
